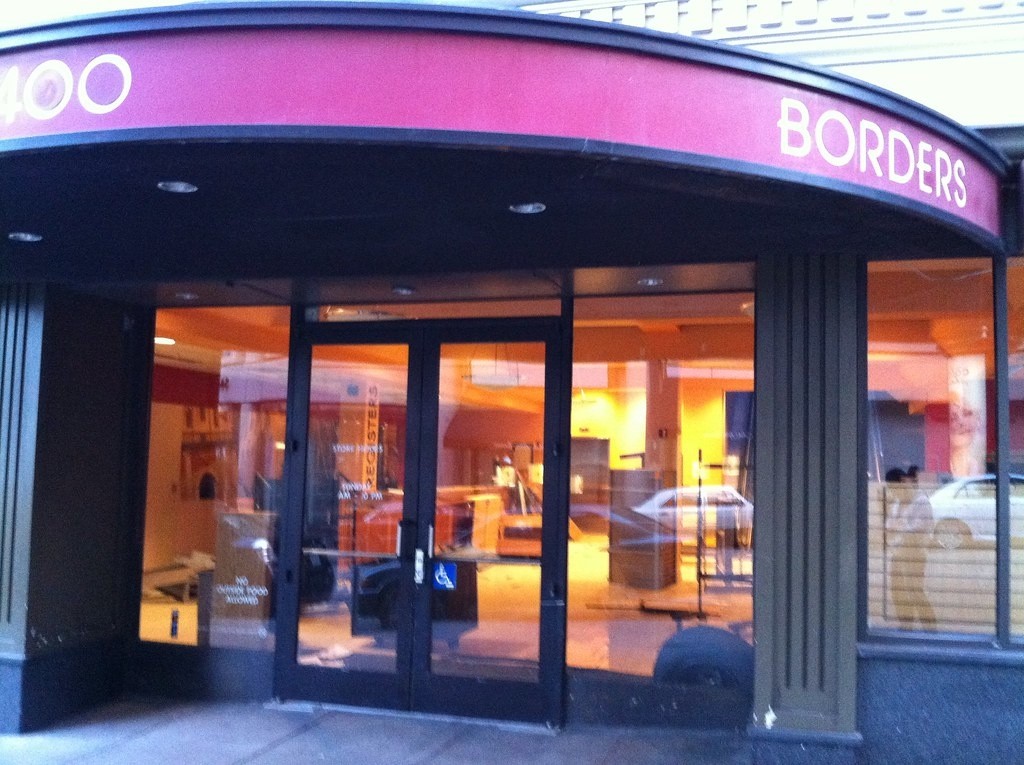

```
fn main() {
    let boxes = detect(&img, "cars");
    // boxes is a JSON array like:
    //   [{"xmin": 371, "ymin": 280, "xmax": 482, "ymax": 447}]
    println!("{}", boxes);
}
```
[
  {"xmin": 927, "ymin": 473, "xmax": 1024, "ymax": 545},
  {"xmin": 636, "ymin": 484, "xmax": 753, "ymax": 530}
]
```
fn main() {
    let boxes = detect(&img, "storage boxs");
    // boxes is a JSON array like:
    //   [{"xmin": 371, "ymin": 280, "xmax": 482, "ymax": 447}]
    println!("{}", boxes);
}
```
[{"xmin": 212, "ymin": 510, "xmax": 277, "ymax": 619}]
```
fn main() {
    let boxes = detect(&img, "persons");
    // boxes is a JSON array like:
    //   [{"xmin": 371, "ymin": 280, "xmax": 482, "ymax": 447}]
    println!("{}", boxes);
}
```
[
  {"xmin": 884, "ymin": 466, "xmax": 936, "ymax": 631},
  {"xmin": 950, "ymin": 392, "xmax": 978, "ymax": 476}
]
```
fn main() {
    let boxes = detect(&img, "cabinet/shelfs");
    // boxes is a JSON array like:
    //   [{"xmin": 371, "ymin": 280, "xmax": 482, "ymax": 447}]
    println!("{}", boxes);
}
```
[{"xmin": 609, "ymin": 469, "xmax": 677, "ymax": 590}]
